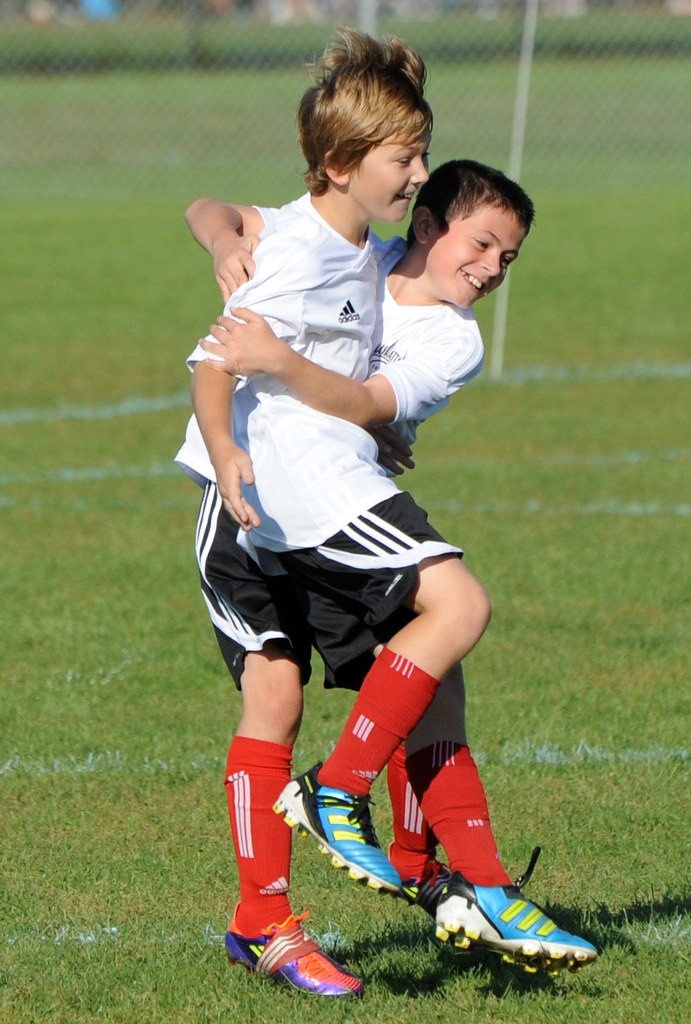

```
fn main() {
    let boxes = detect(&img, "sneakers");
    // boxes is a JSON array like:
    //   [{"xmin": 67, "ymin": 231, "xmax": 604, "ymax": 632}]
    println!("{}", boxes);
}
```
[
  {"xmin": 386, "ymin": 842, "xmax": 452, "ymax": 910},
  {"xmin": 223, "ymin": 903, "xmax": 362, "ymax": 1006},
  {"xmin": 434, "ymin": 870, "xmax": 599, "ymax": 977},
  {"xmin": 272, "ymin": 760, "xmax": 401, "ymax": 895}
]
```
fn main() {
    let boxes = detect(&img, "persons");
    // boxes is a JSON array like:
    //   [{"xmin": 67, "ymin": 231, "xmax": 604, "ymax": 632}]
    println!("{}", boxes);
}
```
[
  {"xmin": 183, "ymin": 158, "xmax": 535, "ymax": 1001},
  {"xmin": 171, "ymin": 29, "xmax": 600, "ymax": 976}
]
